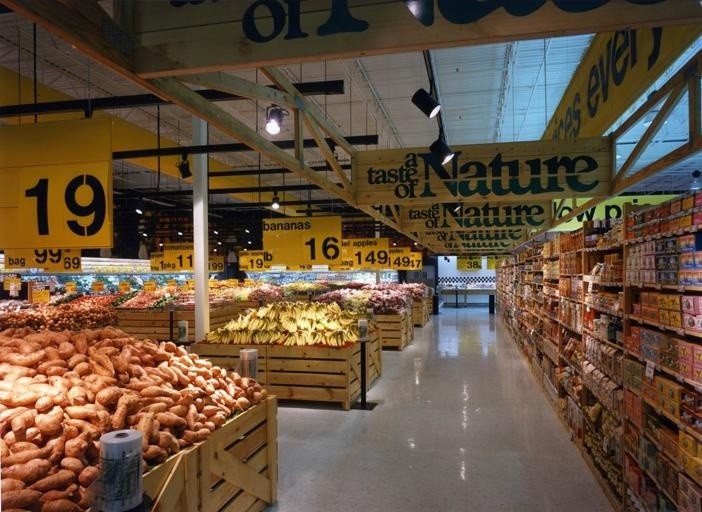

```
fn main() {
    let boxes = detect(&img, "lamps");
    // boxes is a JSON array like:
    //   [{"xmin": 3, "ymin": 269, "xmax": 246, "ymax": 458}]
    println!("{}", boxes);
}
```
[
  {"xmin": 265, "ymin": 103, "xmax": 289, "ymax": 135},
  {"xmin": 176, "ymin": 153, "xmax": 193, "ymax": 180},
  {"xmin": 325, "ymin": 146, "xmax": 339, "ymax": 172},
  {"xmin": 442, "ymin": 202, "xmax": 461, "ymax": 212},
  {"xmin": 411, "ymin": 78, "xmax": 455, "ymax": 167},
  {"xmin": 271, "ymin": 190, "xmax": 280, "ymax": 210}
]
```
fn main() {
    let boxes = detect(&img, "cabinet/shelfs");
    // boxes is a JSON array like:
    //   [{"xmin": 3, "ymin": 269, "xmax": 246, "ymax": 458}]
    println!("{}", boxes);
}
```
[
  {"xmin": 495, "ymin": 187, "xmax": 702, "ymax": 389},
  {"xmin": 542, "ymin": 381, "xmax": 702, "ymax": 510}
]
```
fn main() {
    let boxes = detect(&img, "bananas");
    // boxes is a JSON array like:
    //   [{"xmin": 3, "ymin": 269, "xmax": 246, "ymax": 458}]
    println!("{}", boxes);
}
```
[{"xmin": 201, "ymin": 301, "xmax": 380, "ymax": 349}]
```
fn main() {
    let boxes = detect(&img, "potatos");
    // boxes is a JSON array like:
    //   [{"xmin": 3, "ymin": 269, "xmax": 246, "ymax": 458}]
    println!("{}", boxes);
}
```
[{"xmin": 0, "ymin": 324, "xmax": 266, "ymax": 511}]
